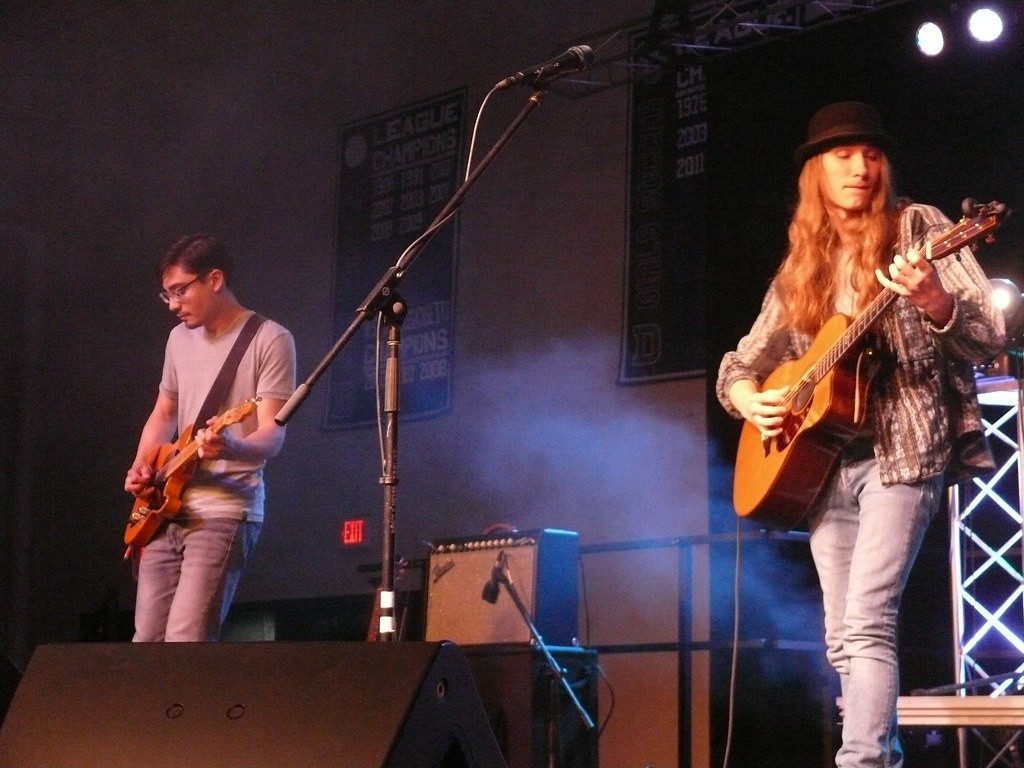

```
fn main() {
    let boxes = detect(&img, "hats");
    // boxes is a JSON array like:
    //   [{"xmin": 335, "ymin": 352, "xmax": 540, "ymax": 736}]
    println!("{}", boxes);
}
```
[{"xmin": 793, "ymin": 100, "xmax": 894, "ymax": 163}]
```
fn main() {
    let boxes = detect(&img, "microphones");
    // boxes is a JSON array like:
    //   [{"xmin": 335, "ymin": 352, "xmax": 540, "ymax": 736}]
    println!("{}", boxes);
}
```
[
  {"xmin": 496, "ymin": 45, "xmax": 595, "ymax": 91},
  {"xmin": 481, "ymin": 551, "xmax": 507, "ymax": 603}
]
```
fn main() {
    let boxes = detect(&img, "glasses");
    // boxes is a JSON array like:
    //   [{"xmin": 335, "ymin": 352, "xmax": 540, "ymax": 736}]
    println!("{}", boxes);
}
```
[{"xmin": 158, "ymin": 266, "xmax": 213, "ymax": 303}]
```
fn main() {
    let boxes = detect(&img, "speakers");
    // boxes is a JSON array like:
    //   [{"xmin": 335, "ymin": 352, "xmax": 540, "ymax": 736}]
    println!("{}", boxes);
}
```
[{"xmin": 0, "ymin": 529, "xmax": 597, "ymax": 768}]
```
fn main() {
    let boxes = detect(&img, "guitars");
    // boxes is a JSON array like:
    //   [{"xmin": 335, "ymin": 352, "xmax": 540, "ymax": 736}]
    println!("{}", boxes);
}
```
[
  {"xmin": 731, "ymin": 196, "xmax": 1015, "ymax": 533},
  {"xmin": 124, "ymin": 395, "xmax": 262, "ymax": 548}
]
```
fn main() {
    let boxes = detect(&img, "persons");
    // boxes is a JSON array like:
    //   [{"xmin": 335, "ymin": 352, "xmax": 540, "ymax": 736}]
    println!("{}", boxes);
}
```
[
  {"xmin": 715, "ymin": 102, "xmax": 1007, "ymax": 768},
  {"xmin": 124, "ymin": 234, "xmax": 297, "ymax": 642}
]
